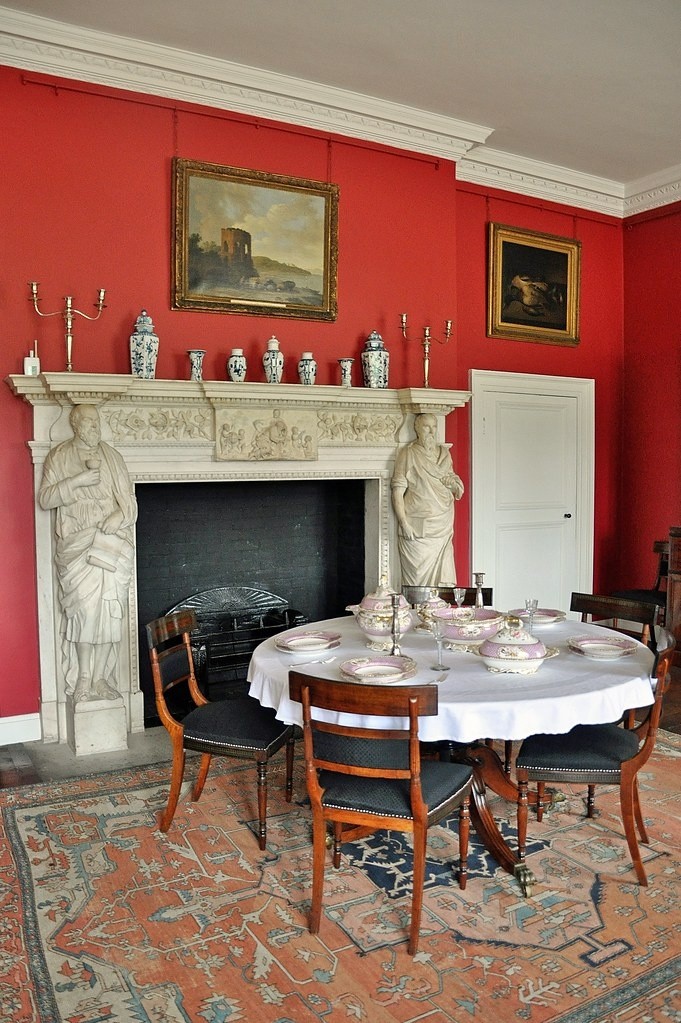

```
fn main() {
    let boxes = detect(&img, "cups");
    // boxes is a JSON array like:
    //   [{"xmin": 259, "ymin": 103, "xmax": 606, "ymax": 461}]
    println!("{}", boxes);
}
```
[
  {"xmin": 187, "ymin": 350, "xmax": 206, "ymax": 381},
  {"xmin": 337, "ymin": 358, "xmax": 355, "ymax": 387}
]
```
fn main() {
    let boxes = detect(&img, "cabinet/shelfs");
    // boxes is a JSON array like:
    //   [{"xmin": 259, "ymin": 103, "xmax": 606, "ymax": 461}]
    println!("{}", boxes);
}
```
[{"xmin": 666, "ymin": 526, "xmax": 681, "ymax": 667}]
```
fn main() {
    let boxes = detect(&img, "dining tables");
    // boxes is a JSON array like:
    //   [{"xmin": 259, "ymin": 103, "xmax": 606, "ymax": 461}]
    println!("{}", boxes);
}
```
[{"xmin": 247, "ymin": 607, "xmax": 656, "ymax": 897}]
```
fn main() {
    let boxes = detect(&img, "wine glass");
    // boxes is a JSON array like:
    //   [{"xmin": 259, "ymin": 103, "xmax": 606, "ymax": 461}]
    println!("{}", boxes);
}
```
[
  {"xmin": 454, "ymin": 588, "xmax": 467, "ymax": 608},
  {"xmin": 429, "ymin": 609, "xmax": 453, "ymax": 671},
  {"xmin": 524, "ymin": 598, "xmax": 538, "ymax": 637}
]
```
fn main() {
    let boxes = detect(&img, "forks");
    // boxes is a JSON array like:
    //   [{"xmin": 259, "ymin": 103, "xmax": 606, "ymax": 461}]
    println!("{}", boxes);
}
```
[{"xmin": 426, "ymin": 673, "xmax": 449, "ymax": 685}]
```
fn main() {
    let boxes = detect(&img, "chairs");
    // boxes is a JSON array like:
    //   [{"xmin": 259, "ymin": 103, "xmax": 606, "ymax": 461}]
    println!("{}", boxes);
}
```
[
  {"xmin": 614, "ymin": 541, "xmax": 669, "ymax": 606},
  {"xmin": 145, "ymin": 585, "xmax": 667, "ymax": 955}
]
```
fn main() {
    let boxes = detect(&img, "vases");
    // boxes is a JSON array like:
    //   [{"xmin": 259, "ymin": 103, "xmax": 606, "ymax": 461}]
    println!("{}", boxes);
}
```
[
  {"xmin": 298, "ymin": 352, "xmax": 317, "ymax": 385},
  {"xmin": 338, "ymin": 358, "xmax": 355, "ymax": 387},
  {"xmin": 487, "ymin": 219, "xmax": 582, "ymax": 348},
  {"xmin": 227, "ymin": 349, "xmax": 247, "ymax": 382},
  {"xmin": 169, "ymin": 154, "xmax": 340, "ymax": 324},
  {"xmin": 187, "ymin": 349, "xmax": 207, "ymax": 380}
]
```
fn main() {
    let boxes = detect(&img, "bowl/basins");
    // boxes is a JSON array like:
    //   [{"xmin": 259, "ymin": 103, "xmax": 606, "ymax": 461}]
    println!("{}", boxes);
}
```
[
  {"xmin": 345, "ymin": 575, "xmax": 413, "ymax": 652},
  {"xmin": 414, "ymin": 589, "xmax": 452, "ymax": 633},
  {"xmin": 432, "ymin": 608, "xmax": 503, "ymax": 651},
  {"xmin": 465, "ymin": 617, "xmax": 560, "ymax": 676}
]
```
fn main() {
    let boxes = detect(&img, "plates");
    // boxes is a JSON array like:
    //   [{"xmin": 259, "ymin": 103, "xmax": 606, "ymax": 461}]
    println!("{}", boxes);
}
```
[
  {"xmin": 566, "ymin": 634, "xmax": 638, "ymax": 662},
  {"xmin": 508, "ymin": 608, "xmax": 566, "ymax": 628},
  {"xmin": 275, "ymin": 631, "xmax": 342, "ymax": 655},
  {"xmin": 339, "ymin": 655, "xmax": 417, "ymax": 685}
]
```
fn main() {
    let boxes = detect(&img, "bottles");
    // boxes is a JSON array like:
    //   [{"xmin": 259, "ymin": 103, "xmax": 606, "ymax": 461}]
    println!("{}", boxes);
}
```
[
  {"xmin": 262, "ymin": 335, "xmax": 284, "ymax": 383},
  {"xmin": 130, "ymin": 310, "xmax": 159, "ymax": 379},
  {"xmin": 298, "ymin": 352, "xmax": 317, "ymax": 385},
  {"xmin": 361, "ymin": 330, "xmax": 389, "ymax": 388},
  {"xmin": 227, "ymin": 348, "xmax": 247, "ymax": 382}
]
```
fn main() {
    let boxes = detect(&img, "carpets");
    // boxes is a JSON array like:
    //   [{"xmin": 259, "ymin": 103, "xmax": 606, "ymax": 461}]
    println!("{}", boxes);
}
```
[{"xmin": 0, "ymin": 718, "xmax": 681, "ymax": 1023}]
